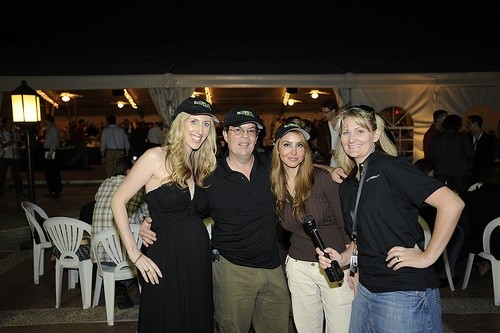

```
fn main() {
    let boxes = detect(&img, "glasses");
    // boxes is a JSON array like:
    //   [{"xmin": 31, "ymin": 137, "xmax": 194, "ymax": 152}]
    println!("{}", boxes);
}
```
[{"xmin": 345, "ymin": 105, "xmax": 375, "ymax": 120}]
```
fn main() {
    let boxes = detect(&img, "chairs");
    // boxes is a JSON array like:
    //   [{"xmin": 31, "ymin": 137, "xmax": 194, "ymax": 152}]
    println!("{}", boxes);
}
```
[
  {"xmin": 417, "ymin": 214, "xmax": 455, "ymax": 291},
  {"xmin": 21, "ymin": 201, "xmax": 143, "ymax": 326},
  {"xmin": 462, "ymin": 218, "xmax": 500, "ymax": 306}
]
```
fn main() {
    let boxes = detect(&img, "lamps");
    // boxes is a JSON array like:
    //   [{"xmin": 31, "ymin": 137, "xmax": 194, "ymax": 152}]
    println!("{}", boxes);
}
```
[
  {"xmin": 59, "ymin": 92, "xmax": 78, "ymax": 103},
  {"xmin": 286, "ymin": 99, "xmax": 303, "ymax": 106},
  {"xmin": 309, "ymin": 90, "xmax": 331, "ymax": 99},
  {"xmin": 110, "ymin": 95, "xmax": 129, "ymax": 108},
  {"xmin": 191, "ymin": 90, "xmax": 205, "ymax": 98}
]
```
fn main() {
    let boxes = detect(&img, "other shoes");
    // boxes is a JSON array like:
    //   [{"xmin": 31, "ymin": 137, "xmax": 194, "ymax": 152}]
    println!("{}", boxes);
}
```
[
  {"xmin": 45, "ymin": 193, "xmax": 62, "ymax": 199},
  {"xmin": 115, "ymin": 283, "xmax": 134, "ymax": 310}
]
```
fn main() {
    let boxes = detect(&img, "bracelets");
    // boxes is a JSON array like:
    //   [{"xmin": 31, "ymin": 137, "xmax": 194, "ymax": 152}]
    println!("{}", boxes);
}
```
[{"xmin": 133, "ymin": 253, "xmax": 144, "ymax": 266}]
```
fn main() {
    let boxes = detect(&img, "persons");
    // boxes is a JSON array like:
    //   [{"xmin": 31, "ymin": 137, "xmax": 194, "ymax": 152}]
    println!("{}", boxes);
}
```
[
  {"xmin": 271, "ymin": 123, "xmax": 358, "ymax": 333},
  {"xmin": 374, "ymin": 110, "xmax": 500, "ymax": 279},
  {"xmin": 139, "ymin": 109, "xmax": 347, "ymax": 333},
  {"xmin": 110, "ymin": 98, "xmax": 219, "ymax": 333},
  {"xmin": 258, "ymin": 103, "xmax": 339, "ymax": 167},
  {"xmin": 0, "ymin": 114, "xmax": 163, "ymax": 199},
  {"xmin": 315, "ymin": 106, "xmax": 465, "ymax": 333},
  {"xmin": 90, "ymin": 157, "xmax": 151, "ymax": 305}
]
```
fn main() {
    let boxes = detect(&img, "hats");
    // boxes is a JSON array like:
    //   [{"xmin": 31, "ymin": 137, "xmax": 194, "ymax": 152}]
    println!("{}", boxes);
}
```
[
  {"xmin": 275, "ymin": 122, "xmax": 310, "ymax": 140},
  {"xmin": 175, "ymin": 97, "xmax": 219, "ymax": 122},
  {"xmin": 223, "ymin": 109, "xmax": 264, "ymax": 130}
]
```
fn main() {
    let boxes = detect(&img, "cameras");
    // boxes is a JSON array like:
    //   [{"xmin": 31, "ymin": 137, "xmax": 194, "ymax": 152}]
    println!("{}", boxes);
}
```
[{"xmin": 349, "ymin": 250, "xmax": 357, "ymax": 273}]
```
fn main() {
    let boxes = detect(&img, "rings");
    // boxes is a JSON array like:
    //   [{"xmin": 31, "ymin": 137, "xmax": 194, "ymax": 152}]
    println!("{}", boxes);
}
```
[
  {"xmin": 397, "ymin": 257, "xmax": 400, "ymax": 262},
  {"xmin": 146, "ymin": 269, "xmax": 150, "ymax": 273}
]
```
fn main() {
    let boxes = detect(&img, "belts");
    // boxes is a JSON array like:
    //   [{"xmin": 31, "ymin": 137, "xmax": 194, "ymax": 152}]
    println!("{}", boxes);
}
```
[{"xmin": 107, "ymin": 149, "xmax": 124, "ymax": 151}]
